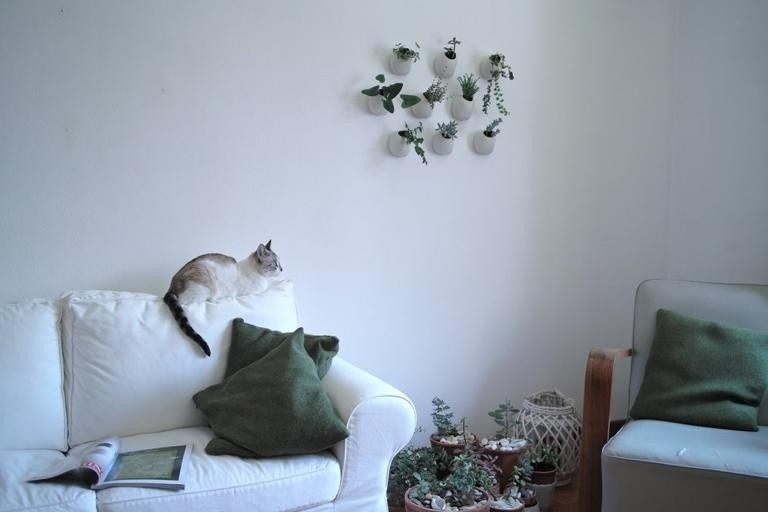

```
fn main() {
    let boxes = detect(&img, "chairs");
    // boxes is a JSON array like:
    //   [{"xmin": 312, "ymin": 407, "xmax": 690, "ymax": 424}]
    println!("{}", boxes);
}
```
[{"xmin": 575, "ymin": 277, "xmax": 767, "ymax": 512}]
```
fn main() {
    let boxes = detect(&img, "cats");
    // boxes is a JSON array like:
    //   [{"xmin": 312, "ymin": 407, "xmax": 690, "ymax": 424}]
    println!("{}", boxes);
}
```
[{"xmin": 163, "ymin": 237, "xmax": 286, "ymax": 359}]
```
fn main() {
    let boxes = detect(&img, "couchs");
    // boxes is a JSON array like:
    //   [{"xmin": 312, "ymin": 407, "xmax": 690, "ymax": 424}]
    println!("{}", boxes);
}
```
[{"xmin": 1, "ymin": 291, "xmax": 418, "ymax": 512}]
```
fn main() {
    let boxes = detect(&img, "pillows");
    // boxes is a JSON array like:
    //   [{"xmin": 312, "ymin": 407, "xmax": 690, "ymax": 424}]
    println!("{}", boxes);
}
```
[
  {"xmin": 626, "ymin": 313, "xmax": 766, "ymax": 431},
  {"xmin": 191, "ymin": 317, "xmax": 349, "ymax": 459}
]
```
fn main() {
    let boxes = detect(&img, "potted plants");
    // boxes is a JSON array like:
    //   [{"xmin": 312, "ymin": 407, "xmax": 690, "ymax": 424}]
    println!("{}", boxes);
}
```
[
  {"xmin": 362, "ymin": 37, "xmax": 514, "ymax": 167},
  {"xmin": 387, "ymin": 396, "xmax": 559, "ymax": 511}
]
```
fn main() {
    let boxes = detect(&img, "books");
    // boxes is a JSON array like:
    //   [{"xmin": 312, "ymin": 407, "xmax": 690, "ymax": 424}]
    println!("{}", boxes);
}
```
[{"xmin": 25, "ymin": 435, "xmax": 195, "ymax": 490}]
